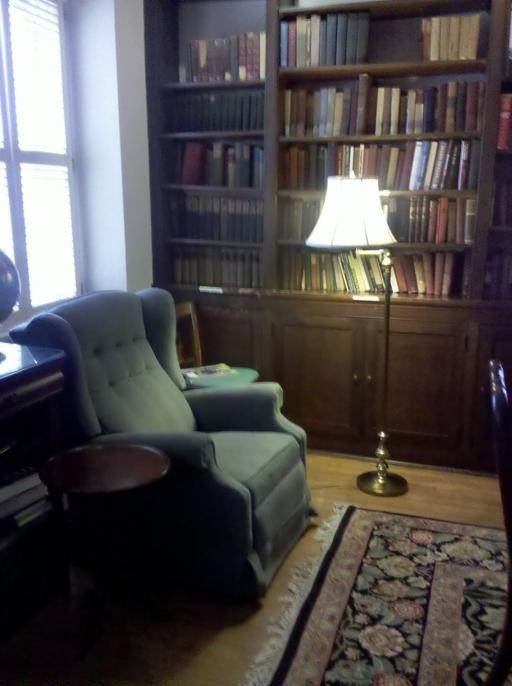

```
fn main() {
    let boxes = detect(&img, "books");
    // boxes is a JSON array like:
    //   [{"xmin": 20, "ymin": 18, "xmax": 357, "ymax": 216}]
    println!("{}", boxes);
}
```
[
  {"xmin": 280, "ymin": 10, "xmax": 370, "ymax": 67},
  {"xmin": 167, "ymin": 30, "xmax": 265, "ymax": 288},
  {"xmin": 277, "ymin": 197, "xmax": 478, "ymax": 297},
  {"xmin": 178, "ymin": 362, "xmax": 238, "ymax": 379},
  {"xmin": 277, "ymin": 73, "xmax": 483, "ymax": 189},
  {"xmin": 419, "ymin": 11, "xmax": 488, "ymax": 62},
  {"xmin": 0, "ymin": 464, "xmax": 62, "ymax": 533}
]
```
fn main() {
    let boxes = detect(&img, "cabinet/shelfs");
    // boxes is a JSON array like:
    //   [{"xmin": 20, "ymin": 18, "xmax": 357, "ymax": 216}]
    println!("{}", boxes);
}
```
[
  {"xmin": 146, "ymin": 0, "xmax": 511, "ymax": 477},
  {"xmin": 1, "ymin": 332, "xmax": 70, "ymax": 651}
]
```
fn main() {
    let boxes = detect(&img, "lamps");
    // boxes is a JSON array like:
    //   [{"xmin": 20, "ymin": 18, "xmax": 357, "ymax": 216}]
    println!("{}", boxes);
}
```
[{"xmin": 304, "ymin": 178, "xmax": 409, "ymax": 497}]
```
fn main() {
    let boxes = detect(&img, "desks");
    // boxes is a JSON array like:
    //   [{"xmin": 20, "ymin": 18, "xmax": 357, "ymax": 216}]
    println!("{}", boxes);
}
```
[{"xmin": 39, "ymin": 440, "xmax": 174, "ymax": 661}]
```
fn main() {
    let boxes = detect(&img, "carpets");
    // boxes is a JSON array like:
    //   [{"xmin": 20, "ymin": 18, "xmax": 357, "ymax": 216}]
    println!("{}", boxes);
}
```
[{"xmin": 247, "ymin": 502, "xmax": 511, "ymax": 684}]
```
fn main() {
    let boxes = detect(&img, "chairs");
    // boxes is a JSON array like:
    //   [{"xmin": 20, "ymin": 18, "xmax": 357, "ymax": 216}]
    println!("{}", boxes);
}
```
[
  {"xmin": 489, "ymin": 360, "xmax": 512, "ymax": 683},
  {"xmin": 10, "ymin": 288, "xmax": 318, "ymax": 611},
  {"xmin": 176, "ymin": 299, "xmax": 261, "ymax": 388}
]
